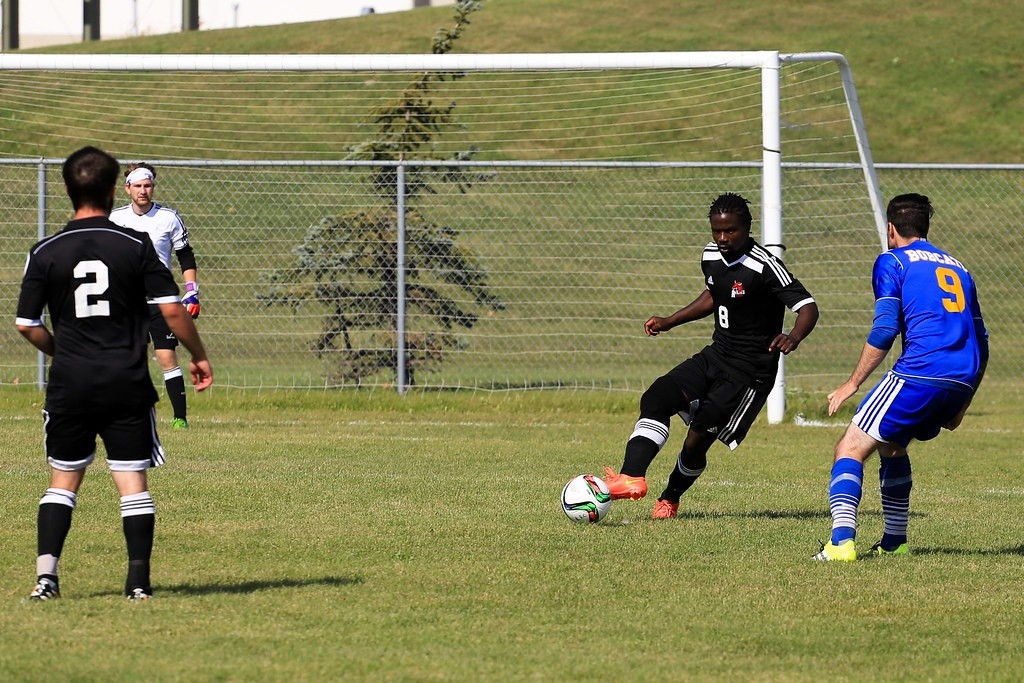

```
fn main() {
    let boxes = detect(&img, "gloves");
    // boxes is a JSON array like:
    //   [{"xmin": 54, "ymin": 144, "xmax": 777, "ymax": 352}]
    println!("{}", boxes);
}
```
[{"xmin": 181, "ymin": 282, "xmax": 201, "ymax": 320}]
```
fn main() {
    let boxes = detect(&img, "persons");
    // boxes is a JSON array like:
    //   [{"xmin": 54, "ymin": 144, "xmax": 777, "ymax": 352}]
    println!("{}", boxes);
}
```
[
  {"xmin": 808, "ymin": 193, "xmax": 990, "ymax": 560},
  {"xmin": 15, "ymin": 145, "xmax": 214, "ymax": 603},
  {"xmin": 107, "ymin": 162, "xmax": 202, "ymax": 429},
  {"xmin": 600, "ymin": 193, "xmax": 820, "ymax": 519}
]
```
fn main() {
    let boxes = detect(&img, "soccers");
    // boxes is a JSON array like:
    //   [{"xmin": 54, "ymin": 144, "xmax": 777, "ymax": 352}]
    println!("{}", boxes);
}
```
[{"xmin": 560, "ymin": 474, "xmax": 612, "ymax": 523}]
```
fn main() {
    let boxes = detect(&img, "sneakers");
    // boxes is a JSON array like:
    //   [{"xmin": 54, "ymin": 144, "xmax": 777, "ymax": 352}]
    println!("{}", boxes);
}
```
[
  {"xmin": 650, "ymin": 498, "xmax": 680, "ymax": 522},
  {"xmin": 811, "ymin": 536, "xmax": 857, "ymax": 560},
  {"xmin": 170, "ymin": 417, "xmax": 188, "ymax": 429},
  {"xmin": 29, "ymin": 580, "xmax": 62, "ymax": 602},
  {"xmin": 861, "ymin": 541, "xmax": 912, "ymax": 560},
  {"xmin": 603, "ymin": 474, "xmax": 648, "ymax": 500},
  {"xmin": 125, "ymin": 585, "xmax": 153, "ymax": 600}
]
